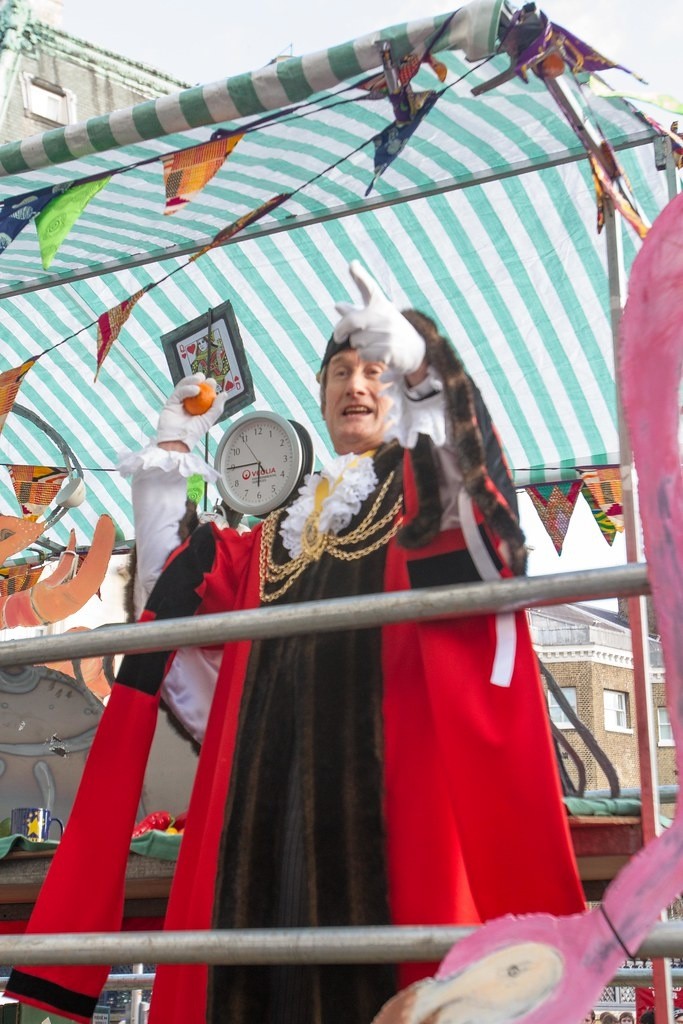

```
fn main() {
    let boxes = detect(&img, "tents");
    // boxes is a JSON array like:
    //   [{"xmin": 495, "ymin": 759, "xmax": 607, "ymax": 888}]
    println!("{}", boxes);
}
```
[{"xmin": 0, "ymin": 0, "xmax": 683, "ymax": 851}]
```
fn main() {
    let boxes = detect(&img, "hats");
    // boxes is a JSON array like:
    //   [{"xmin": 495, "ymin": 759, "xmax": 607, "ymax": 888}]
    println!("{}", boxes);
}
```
[{"xmin": 319, "ymin": 333, "xmax": 350, "ymax": 366}]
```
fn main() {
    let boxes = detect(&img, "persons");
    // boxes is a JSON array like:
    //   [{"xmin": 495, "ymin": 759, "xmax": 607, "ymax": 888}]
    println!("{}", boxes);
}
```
[{"xmin": 117, "ymin": 260, "xmax": 588, "ymax": 1024}]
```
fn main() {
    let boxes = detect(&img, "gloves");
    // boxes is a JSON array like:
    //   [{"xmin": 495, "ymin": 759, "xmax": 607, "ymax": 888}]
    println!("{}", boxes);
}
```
[
  {"xmin": 157, "ymin": 371, "xmax": 228, "ymax": 452},
  {"xmin": 333, "ymin": 257, "xmax": 426, "ymax": 378}
]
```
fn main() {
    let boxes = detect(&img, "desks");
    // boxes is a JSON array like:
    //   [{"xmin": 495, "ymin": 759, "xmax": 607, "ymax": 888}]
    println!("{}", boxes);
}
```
[{"xmin": 0, "ymin": 798, "xmax": 645, "ymax": 1024}]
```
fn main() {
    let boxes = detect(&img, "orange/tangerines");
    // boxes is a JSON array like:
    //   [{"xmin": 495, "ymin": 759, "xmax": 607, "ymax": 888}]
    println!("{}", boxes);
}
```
[{"xmin": 183, "ymin": 383, "xmax": 216, "ymax": 415}]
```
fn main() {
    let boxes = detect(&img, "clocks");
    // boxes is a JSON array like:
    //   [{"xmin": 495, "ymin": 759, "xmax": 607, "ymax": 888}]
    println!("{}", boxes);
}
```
[{"xmin": 215, "ymin": 410, "xmax": 315, "ymax": 518}]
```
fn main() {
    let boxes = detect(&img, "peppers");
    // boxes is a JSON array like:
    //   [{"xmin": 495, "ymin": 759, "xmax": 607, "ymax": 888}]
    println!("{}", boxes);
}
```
[{"xmin": 132, "ymin": 812, "xmax": 175, "ymax": 838}]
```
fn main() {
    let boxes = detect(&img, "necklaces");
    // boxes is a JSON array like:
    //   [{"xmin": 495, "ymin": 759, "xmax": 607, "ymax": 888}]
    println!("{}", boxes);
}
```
[{"xmin": 258, "ymin": 448, "xmax": 404, "ymax": 602}]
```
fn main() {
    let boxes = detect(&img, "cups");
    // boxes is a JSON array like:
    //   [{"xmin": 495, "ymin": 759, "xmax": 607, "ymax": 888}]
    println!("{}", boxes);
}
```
[{"xmin": 7, "ymin": 808, "xmax": 64, "ymax": 842}]
